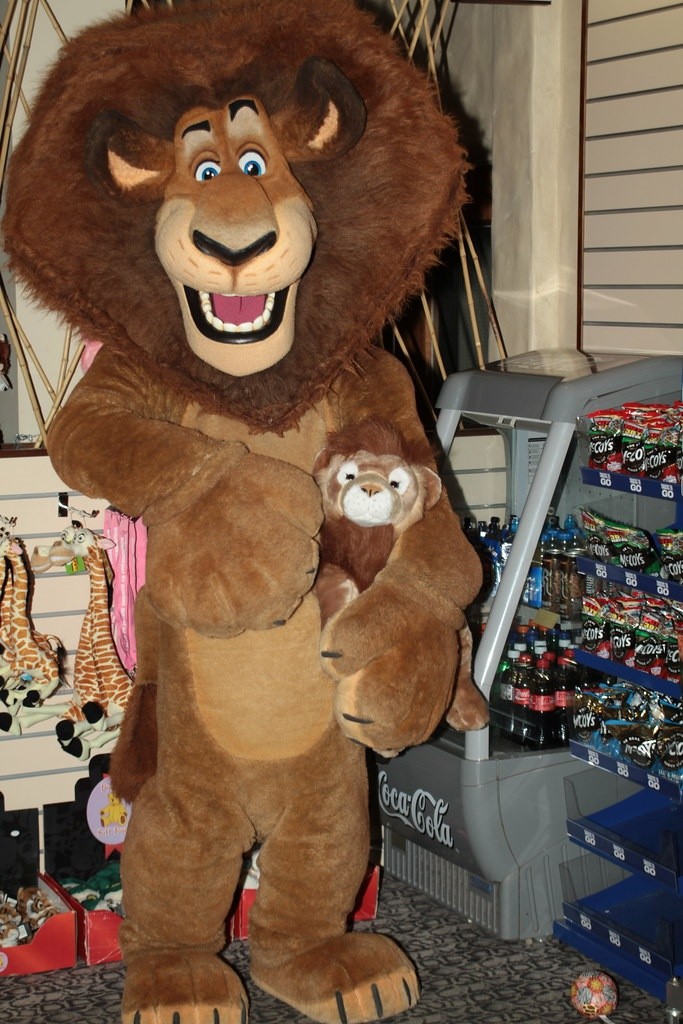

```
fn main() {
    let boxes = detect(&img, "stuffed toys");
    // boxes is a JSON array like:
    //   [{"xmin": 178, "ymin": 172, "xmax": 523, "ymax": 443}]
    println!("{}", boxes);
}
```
[
  {"xmin": 311, "ymin": 416, "xmax": 491, "ymax": 731},
  {"xmin": 47, "ymin": 520, "xmax": 141, "ymax": 762},
  {"xmin": 0, "ymin": 757, "xmax": 135, "ymax": 950},
  {"xmin": 0, "ymin": 532, "xmax": 67, "ymax": 736},
  {"xmin": 2, "ymin": 0, "xmax": 483, "ymax": 1024}
]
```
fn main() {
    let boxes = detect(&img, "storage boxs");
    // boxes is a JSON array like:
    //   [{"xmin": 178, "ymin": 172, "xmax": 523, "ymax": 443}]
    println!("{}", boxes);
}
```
[{"xmin": 0, "ymin": 843, "xmax": 380, "ymax": 976}]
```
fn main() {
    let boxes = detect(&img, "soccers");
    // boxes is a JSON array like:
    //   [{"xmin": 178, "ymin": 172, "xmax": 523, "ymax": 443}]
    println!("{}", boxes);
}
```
[{"xmin": 570, "ymin": 971, "xmax": 618, "ymax": 1021}]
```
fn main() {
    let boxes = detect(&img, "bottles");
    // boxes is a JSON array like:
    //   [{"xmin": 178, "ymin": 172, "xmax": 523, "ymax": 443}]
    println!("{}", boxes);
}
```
[
  {"xmin": 481, "ymin": 618, "xmax": 576, "ymax": 751},
  {"xmin": 462, "ymin": 514, "xmax": 582, "ymax": 607}
]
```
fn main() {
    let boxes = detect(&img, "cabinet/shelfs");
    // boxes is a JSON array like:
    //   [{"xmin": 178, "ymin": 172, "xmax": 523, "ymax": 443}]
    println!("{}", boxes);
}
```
[
  {"xmin": 550, "ymin": 463, "xmax": 683, "ymax": 1024},
  {"xmin": 373, "ymin": 348, "xmax": 683, "ymax": 940}
]
